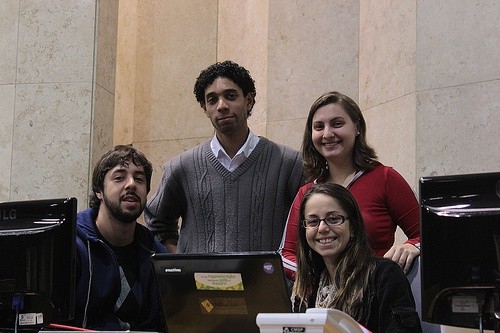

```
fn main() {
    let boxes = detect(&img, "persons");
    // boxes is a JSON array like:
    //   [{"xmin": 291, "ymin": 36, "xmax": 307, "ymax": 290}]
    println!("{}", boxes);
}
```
[
  {"xmin": 278, "ymin": 91, "xmax": 420, "ymax": 280},
  {"xmin": 73, "ymin": 143, "xmax": 169, "ymax": 333},
  {"xmin": 143, "ymin": 60, "xmax": 311, "ymax": 254},
  {"xmin": 285, "ymin": 183, "xmax": 422, "ymax": 332}
]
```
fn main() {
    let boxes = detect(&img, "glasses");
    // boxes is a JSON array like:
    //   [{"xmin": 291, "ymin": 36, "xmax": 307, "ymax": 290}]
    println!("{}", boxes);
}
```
[{"xmin": 303, "ymin": 214, "xmax": 351, "ymax": 228}]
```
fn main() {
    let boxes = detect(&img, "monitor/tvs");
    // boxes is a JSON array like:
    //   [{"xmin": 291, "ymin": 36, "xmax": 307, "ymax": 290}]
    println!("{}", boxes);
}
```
[
  {"xmin": 420, "ymin": 172, "xmax": 500, "ymax": 333},
  {"xmin": 0, "ymin": 197, "xmax": 77, "ymax": 333}
]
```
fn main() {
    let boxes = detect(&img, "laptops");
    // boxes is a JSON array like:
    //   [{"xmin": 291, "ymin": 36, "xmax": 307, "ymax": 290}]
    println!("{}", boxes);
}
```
[{"xmin": 149, "ymin": 251, "xmax": 292, "ymax": 333}]
